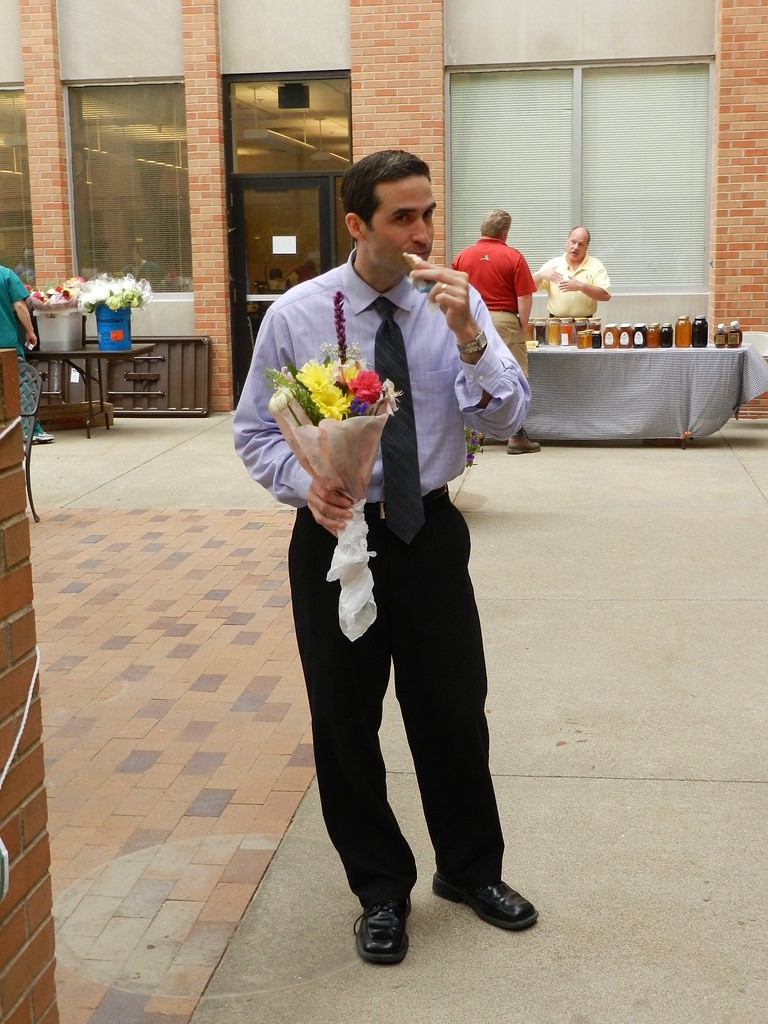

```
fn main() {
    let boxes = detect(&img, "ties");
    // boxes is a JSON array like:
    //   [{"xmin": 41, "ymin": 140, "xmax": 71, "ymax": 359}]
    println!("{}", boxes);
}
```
[{"xmin": 373, "ymin": 297, "xmax": 425, "ymax": 545}]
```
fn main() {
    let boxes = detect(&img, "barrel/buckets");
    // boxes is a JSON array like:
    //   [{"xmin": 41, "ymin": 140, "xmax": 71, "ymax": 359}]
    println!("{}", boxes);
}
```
[
  {"xmin": 95, "ymin": 304, "xmax": 132, "ymax": 351},
  {"xmin": 13, "ymin": 311, "xmax": 36, "ymax": 350},
  {"xmin": 82, "ymin": 315, "xmax": 86, "ymax": 348}
]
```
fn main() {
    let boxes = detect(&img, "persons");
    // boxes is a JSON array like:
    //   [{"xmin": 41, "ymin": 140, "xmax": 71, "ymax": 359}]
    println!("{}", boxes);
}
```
[
  {"xmin": 269, "ymin": 260, "xmax": 319, "ymax": 294},
  {"xmin": 531, "ymin": 226, "xmax": 611, "ymax": 320},
  {"xmin": 119, "ymin": 239, "xmax": 168, "ymax": 292},
  {"xmin": 0, "ymin": 260, "xmax": 56, "ymax": 443},
  {"xmin": 449, "ymin": 209, "xmax": 541, "ymax": 455},
  {"xmin": 233, "ymin": 149, "xmax": 539, "ymax": 964},
  {"xmin": 11, "ymin": 246, "xmax": 35, "ymax": 286}
]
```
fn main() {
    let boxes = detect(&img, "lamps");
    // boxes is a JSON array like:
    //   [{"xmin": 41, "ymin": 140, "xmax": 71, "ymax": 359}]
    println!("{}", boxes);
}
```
[
  {"xmin": 278, "ymin": 82, "xmax": 311, "ymax": 109},
  {"xmin": 265, "ymin": 110, "xmax": 317, "ymax": 150},
  {"xmin": 329, "ymin": 151, "xmax": 349, "ymax": 162},
  {"xmin": 310, "ymin": 116, "xmax": 332, "ymax": 162},
  {"xmin": 146, "ymin": 141, "xmax": 188, "ymax": 170},
  {"xmin": 242, "ymin": 86, "xmax": 270, "ymax": 140},
  {"xmin": 3, "ymin": 92, "xmax": 27, "ymax": 146},
  {"xmin": 83, "ymin": 120, "xmax": 146, "ymax": 163},
  {"xmin": 0, "ymin": 147, "xmax": 23, "ymax": 175}
]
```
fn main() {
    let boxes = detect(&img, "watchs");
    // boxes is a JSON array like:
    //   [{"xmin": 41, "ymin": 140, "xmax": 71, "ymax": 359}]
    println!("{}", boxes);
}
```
[{"xmin": 456, "ymin": 329, "xmax": 487, "ymax": 355}]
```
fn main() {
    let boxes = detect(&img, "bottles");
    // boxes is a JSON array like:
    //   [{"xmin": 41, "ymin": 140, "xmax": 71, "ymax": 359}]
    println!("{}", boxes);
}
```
[
  {"xmin": 714, "ymin": 321, "xmax": 743, "ymax": 348},
  {"xmin": 675, "ymin": 315, "xmax": 708, "ymax": 348},
  {"xmin": 603, "ymin": 323, "xmax": 674, "ymax": 349},
  {"xmin": 524, "ymin": 317, "xmax": 602, "ymax": 349}
]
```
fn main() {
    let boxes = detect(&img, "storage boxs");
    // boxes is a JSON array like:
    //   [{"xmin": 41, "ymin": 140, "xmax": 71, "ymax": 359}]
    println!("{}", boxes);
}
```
[
  {"xmin": 36, "ymin": 400, "xmax": 114, "ymax": 432},
  {"xmin": 32, "ymin": 309, "xmax": 83, "ymax": 352}
]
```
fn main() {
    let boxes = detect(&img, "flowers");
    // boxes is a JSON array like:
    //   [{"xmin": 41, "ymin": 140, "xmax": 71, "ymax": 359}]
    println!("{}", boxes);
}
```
[
  {"xmin": 464, "ymin": 425, "xmax": 481, "ymax": 469},
  {"xmin": 24, "ymin": 276, "xmax": 86, "ymax": 311},
  {"xmin": 259, "ymin": 289, "xmax": 405, "ymax": 638},
  {"xmin": 76, "ymin": 271, "xmax": 155, "ymax": 315}
]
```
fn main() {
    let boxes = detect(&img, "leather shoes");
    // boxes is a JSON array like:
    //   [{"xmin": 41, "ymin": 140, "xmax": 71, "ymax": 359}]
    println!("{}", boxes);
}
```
[
  {"xmin": 356, "ymin": 894, "xmax": 411, "ymax": 964},
  {"xmin": 432, "ymin": 873, "xmax": 539, "ymax": 928}
]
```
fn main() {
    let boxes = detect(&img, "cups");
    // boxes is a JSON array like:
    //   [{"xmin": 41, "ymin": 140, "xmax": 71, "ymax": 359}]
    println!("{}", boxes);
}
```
[{"xmin": 269, "ymin": 281, "xmax": 286, "ymax": 290}]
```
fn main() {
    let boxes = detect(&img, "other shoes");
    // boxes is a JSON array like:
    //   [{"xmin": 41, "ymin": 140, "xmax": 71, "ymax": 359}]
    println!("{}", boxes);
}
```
[{"xmin": 508, "ymin": 434, "xmax": 540, "ymax": 453}]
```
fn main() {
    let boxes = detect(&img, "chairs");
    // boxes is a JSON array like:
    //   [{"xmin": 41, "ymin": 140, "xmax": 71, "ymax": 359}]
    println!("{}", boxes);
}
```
[{"xmin": 18, "ymin": 363, "xmax": 43, "ymax": 523}]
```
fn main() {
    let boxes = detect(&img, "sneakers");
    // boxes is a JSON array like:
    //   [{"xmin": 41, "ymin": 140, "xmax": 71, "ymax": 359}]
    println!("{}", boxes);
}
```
[{"xmin": 26, "ymin": 432, "xmax": 54, "ymax": 442}]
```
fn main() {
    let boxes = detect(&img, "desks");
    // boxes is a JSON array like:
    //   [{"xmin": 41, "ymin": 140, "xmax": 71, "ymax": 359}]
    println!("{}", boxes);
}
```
[
  {"xmin": 529, "ymin": 343, "xmax": 768, "ymax": 451},
  {"xmin": 23, "ymin": 343, "xmax": 157, "ymax": 440}
]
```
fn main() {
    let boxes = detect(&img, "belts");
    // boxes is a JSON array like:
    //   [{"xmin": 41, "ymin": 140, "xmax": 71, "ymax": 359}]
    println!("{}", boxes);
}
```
[
  {"xmin": 365, "ymin": 482, "xmax": 448, "ymax": 517},
  {"xmin": 550, "ymin": 313, "xmax": 592, "ymax": 318}
]
